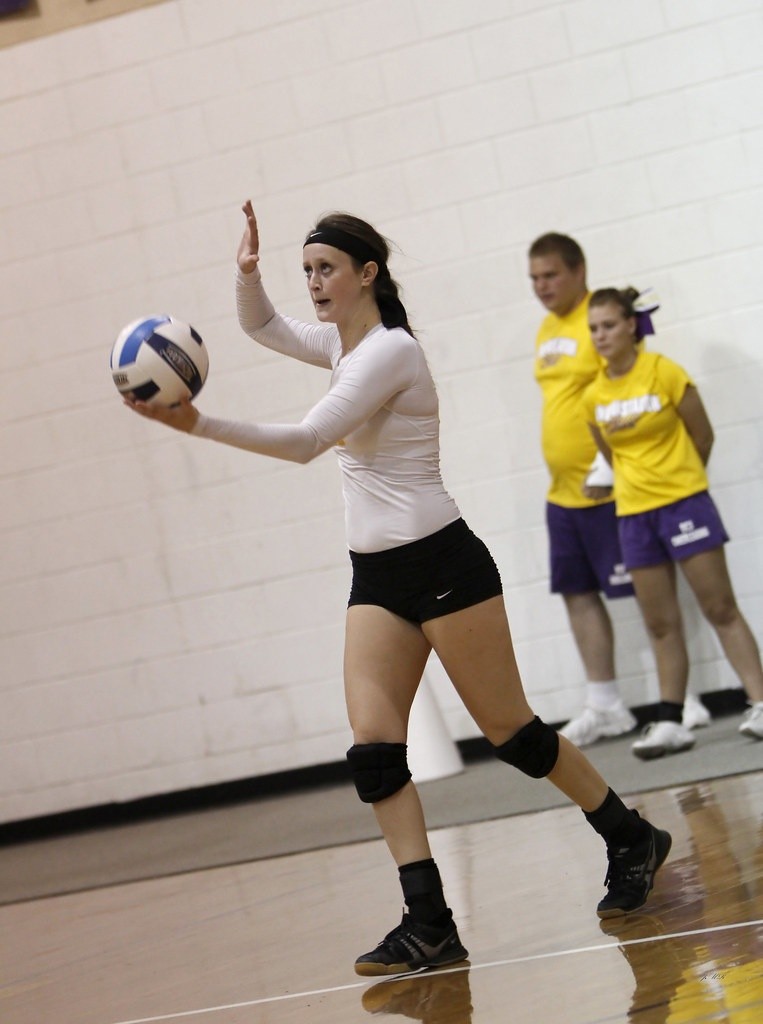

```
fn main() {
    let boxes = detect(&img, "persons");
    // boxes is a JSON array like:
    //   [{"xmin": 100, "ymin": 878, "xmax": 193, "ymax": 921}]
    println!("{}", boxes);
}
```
[
  {"xmin": 122, "ymin": 200, "xmax": 673, "ymax": 977},
  {"xmin": 526, "ymin": 232, "xmax": 711, "ymax": 749},
  {"xmin": 582, "ymin": 287, "xmax": 763, "ymax": 761}
]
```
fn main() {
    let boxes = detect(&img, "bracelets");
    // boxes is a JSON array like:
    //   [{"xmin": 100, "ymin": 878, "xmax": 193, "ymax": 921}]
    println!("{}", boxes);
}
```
[{"xmin": 235, "ymin": 264, "xmax": 262, "ymax": 284}]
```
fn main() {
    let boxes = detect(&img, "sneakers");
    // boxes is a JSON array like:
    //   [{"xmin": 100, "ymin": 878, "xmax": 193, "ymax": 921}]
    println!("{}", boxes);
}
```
[
  {"xmin": 680, "ymin": 684, "xmax": 712, "ymax": 731},
  {"xmin": 593, "ymin": 809, "xmax": 671, "ymax": 919},
  {"xmin": 556, "ymin": 702, "xmax": 638, "ymax": 749},
  {"xmin": 634, "ymin": 709, "xmax": 698, "ymax": 760},
  {"xmin": 738, "ymin": 697, "xmax": 763, "ymax": 739},
  {"xmin": 354, "ymin": 906, "xmax": 464, "ymax": 973},
  {"xmin": 361, "ymin": 959, "xmax": 475, "ymax": 1019}
]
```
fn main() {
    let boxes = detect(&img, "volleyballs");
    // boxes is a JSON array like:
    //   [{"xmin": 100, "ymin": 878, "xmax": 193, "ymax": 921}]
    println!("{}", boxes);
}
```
[{"xmin": 110, "ymin": 314, "xmax": 209, "ymax": 408}]
